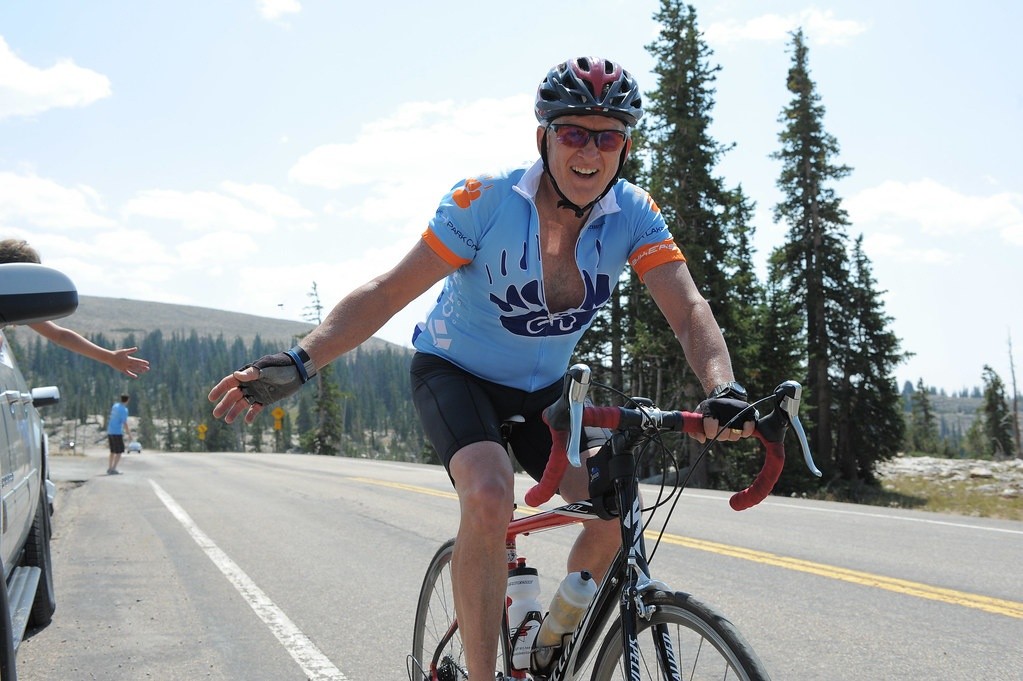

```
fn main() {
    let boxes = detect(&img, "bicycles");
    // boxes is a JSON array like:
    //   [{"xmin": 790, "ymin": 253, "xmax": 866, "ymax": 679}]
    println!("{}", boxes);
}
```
[{"xmin": 403, "ymin": 361, "xmax": 823, "ymax": 681}]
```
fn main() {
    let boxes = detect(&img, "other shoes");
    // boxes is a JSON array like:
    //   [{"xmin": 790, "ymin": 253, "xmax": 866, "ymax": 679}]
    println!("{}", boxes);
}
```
[{"xmin": 107, "ymin": 468, "xmax": 123, "ymax": 475}]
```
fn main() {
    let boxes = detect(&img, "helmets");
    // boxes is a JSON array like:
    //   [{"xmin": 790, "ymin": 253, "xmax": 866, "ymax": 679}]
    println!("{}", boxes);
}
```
[{"xmin": 535, "ymin": 56, "xmax": 643, "ymax": 128}]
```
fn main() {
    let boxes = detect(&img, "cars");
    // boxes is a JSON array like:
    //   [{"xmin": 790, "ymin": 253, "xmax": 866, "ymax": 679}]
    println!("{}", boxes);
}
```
[
  {"xmin": 0, "ymin": 261, "xmax": 80, "ymax": 681},
  {"xmin": 127, "ymin": 442, "xmax": 142, "ymax": 453}
]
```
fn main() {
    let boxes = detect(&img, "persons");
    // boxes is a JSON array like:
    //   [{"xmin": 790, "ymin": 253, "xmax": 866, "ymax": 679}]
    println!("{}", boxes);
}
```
[
  {"xmin": 106, "ymin": 392, "xmax": 134, "ymax": 476},
  {"xmin": 0, "ymin": 236, "xmax": 151, "ymax": 379},
  {"xmin": 207, "ymin": 54, "xmax": 756, "ymax": 681}
]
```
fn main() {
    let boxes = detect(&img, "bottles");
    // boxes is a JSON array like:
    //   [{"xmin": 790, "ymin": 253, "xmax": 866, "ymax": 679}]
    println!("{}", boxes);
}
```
[
  {"xmin": 530, "ymin": 569, "xmax": 597, "ymax": 676},
  {"xmin": 505, "ymin": 557, "xmax": 542, "ymax": 670}
]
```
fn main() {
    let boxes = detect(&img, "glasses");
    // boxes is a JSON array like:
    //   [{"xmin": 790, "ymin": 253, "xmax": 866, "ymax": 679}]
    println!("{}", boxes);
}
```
[{"xmin": 548, "ymin": 123, "xmax": 628, "ymax": 153}]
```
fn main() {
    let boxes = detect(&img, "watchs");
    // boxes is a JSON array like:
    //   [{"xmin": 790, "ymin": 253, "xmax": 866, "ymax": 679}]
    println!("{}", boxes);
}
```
[{"xmin": 703, "ymin": 380, "xmax": 749, "ymax": 401}]
